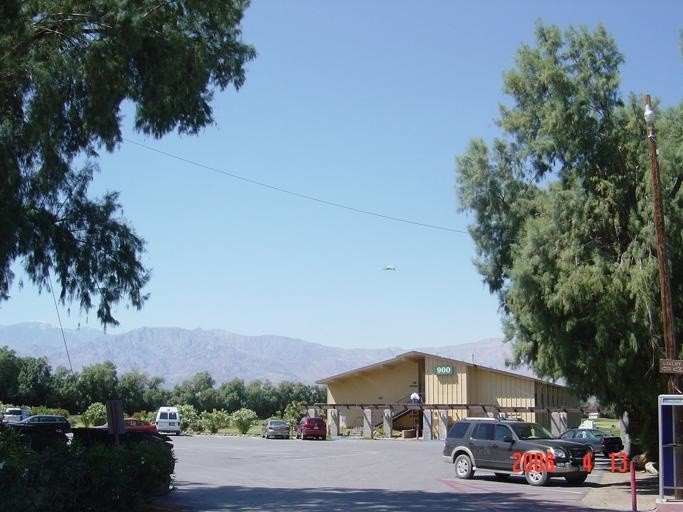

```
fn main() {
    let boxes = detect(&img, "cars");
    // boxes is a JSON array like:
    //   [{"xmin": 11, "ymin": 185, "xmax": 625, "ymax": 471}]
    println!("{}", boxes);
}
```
[
  {"xmin": 295, "ymin": 417, "xmax": 327, "ymax": 441},
  {"xmin": 555, "ymin": 426, "xmax": 625, "ymax": 459},
  {"xmin": 261, "ymin": 419, "xmax": 291, "ymax": 439},
  {"xmin": 8, "ymin": 413, "xmax": 72, "ymax": 434},
  {"xmin": 94, "ymin": 417, "xmax": 159, "ymax": 435}
]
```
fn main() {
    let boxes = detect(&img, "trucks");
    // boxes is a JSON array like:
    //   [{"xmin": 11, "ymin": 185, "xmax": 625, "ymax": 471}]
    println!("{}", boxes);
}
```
[{"xmin": 154, "ymin": 406, "xmax": 182, "ymax": 436}]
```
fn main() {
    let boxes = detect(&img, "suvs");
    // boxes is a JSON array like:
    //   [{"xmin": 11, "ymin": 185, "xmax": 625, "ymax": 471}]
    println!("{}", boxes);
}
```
[{"xmin": 441, "ymin": 414, "xmax": 597, "ymax": 488}]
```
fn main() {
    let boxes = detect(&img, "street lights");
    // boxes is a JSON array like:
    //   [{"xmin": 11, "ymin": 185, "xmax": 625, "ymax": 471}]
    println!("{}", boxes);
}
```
[{"xmin": 641, "ymin": 90, "xmax": 681, "ymax": 394}]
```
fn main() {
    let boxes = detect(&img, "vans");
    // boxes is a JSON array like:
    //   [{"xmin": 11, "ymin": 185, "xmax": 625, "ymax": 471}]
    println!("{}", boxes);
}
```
[{"xmin": 2, "ymin": 407, "xmax": 29, "ymax": 426}]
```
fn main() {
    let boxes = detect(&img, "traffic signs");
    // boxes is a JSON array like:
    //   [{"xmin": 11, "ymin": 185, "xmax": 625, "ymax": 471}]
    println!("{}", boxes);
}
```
[{"xmin": 659, "ymin": 359, "xmax": 683, "ymax": 374}]
